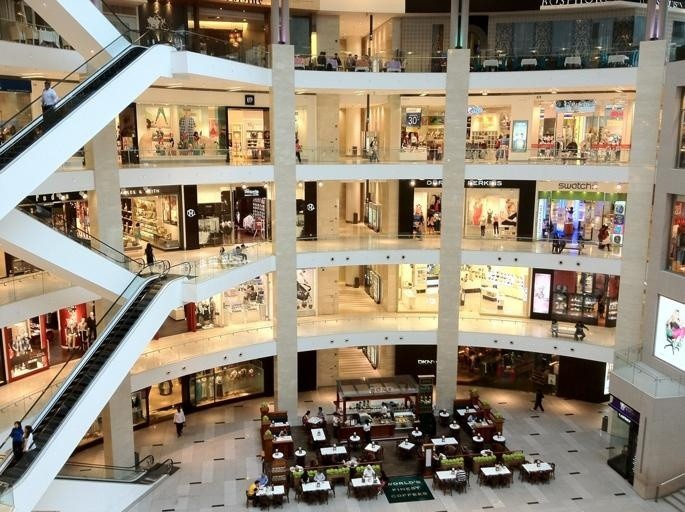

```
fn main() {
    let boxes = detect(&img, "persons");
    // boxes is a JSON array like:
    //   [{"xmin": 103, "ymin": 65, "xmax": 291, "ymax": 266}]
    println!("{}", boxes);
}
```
[
  {"xmin": 10, "ymin": 420, "xmax": 36, "ymax": 468},
  {"xmin": 173, "ymin": 405, "xmax": 185, "ymax": 437},
  {"xmin": 144, "ymin": 243, "xmax": 157, "ymax": 276},
  {"xmin": 317, "ymin": 51, "xmax": 406, "ymax": 75},
  {"xmin": 179, "ymin": 107, "xmax": 197, "ymax": 142},
  {"xmin": 551, "ymin": 319, "xmax": 589, "ymax": 341},
  {"xmin": 65, "ymin": 312, "xmax": 97, "ymax": 353},
  {"xmin": 41, "ymin": 80, "xmax": 59, "ymax": 120},
  {"xmin": 479, "ymin": 209, "xmax": 499, "ymax": 237},
  {"xmin": 7, "ymin": 334, "xmax": 33, "ymax": 372},
  {"xmin": 279, "ymin": 428, "xmax": 288, "ymax": 436},
  {"xmin": 370, "ymin": 137, "xmax": 380, "ymax": 162},
  {"xmin": 495, "ymin": 134, "xmax": 509, "ymax": 158},
  {"xmin": 247, "ymin": 473, "xmax": 269, "ymax": 506},
  {"xmin": 196, "ymin": 373, "xmax": 230, "ymax": 403},
  {"xmin": 301, "ymin": 469, "xmax": 326, "ymax": 484},
  {"xmin": 410, "ymin": 132, "xmax": 418, "ymax": 152},
  {"xmin": 380, "ymin": 402, "xmax": 387, "ymax": 415},
  {"xmin": 455, "ymin": 467, "xmax": 467, "ymax": 493},
  {"xmin": 363, "ymin": 419, "xmax": 372, "ymax": 445},
  {"xmin": 147, "ymin": 13, "xmax": 161, "ymax": 45},
  {"xmin": 530, "ymin": 383, "xmax": 545, "ymax": 414},
  {"xmin": 216, "ymin": 247, "xmax": 225, "ymax": 269},
  {"xmin": 363, "ymin": 464, "xmax": 376, "ymax": 477},
  {"xmin": 295, "ymin": 139, "xmax": 303, "ymax": 163},
  {"xmin": 668, "ymin": 309, "xmax": 685, "ymax": 349},
  {"xmin": 597, "ymin": 226, "xmax": 612, "ymax": 251},
  {"xmin": 302, "ymin": 407, "xmax": 341, "ymax": 439},
  {"xmin": 233, "ymin": 243, "xmax": 248, "ymax": 264}
]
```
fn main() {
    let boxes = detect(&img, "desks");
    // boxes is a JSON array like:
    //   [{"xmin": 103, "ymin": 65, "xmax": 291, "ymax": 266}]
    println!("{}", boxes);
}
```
[
  {"xmin": 519, "ymin": 58, "xmax": 537, "ymax": 70},
  {"xmin": 480, "ymin": 57, "xmax": 504, "ymax": 71},
  {"xmin": 518, "ymin": 459, "xmax": 555, "ymax": 485},
  {"xmin": 292, "ymin": 54, "xmax": 409, "ymax": 74},
  {"xmin": 604, "ymin": 52, "xmax": 631, "ymax": 67},
  {"xmin": 562, "ymin": 54, "xmax": 583, "ymax": 70},
  {"xmin": 246, "ymin": 417, "xmax": 381, "ymax": 509},
  {"xmin": 241, "ymin": 399, "xmax": 557, "ymax": 512},
  {"xmin": 398, "ymin": 406, "xmax": 514, "ymax": 496}
]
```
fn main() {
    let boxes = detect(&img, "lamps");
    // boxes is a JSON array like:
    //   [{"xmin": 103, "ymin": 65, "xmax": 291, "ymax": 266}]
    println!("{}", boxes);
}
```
[{"xmin": 34, "ymin": 190, "xmax": 90, "ymax": 203}]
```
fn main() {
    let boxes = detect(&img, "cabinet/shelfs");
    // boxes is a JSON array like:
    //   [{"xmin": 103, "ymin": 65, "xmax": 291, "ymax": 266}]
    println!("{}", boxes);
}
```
[
  {"xmin": 251, "ymin": 197, "xmax": 270, "ymax": 240},
  {"xmin": 131, "ymin": 198, "xmax": 158, "ymax": 241},
  {"xmin": 230, "ymin": 122, "xmax": 244, "ymax": 158},
  {"xmin": 224, "ymin": 276, "xmax": 266, "ymax": 324},
  {"xmin": 413, "ymin": 263, "xmax": 426, "ymax": 294},
  {"xmin": 473, "ymin": 129, "xmax": 497, "ymax": 155},
  {"xmin": 244, "ymin": 130, "xmax": 271, "ymax": 164},
  {"xmin": 120, "ymin": 200, "xmax": 133, "ymax": 237},
  {"xmin": 552, "ymin": 289, "xmax": 600, "ymax": 326},
  {"xmin": 604, "ymin": 297, "xmax": 618, "ymax": 328}
]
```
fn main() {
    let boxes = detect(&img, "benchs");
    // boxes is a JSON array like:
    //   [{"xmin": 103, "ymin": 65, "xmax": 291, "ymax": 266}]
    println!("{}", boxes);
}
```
[
  {"xmin": 552, "ymin": 242, "xmax": 583, "ymax": 254},
  {"xmin": 558, "ymin": 155, "xmax": 590, "ymax": 165}
]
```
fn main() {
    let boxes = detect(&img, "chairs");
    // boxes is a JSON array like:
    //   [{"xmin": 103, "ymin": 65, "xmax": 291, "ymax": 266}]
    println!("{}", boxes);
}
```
[{"xmin": 0, "ymin": 17, "xmax": 75, "ymax": 50}]
